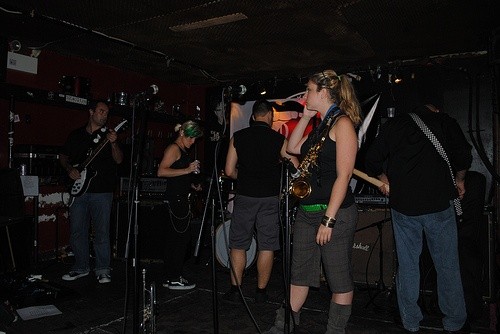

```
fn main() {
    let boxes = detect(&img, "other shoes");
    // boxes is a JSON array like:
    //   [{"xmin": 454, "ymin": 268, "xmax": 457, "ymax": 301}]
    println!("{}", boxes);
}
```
[
  {"xmin": 255, "ymin": 291, "xmax": 268, "ymax": 303},
  {"xmin": 395, "ymin": 318, "xmax": 420, "ymax": 334},
  {"xmin": 221, "ymin": 289, "xmax": 244, "ymax": 304},
  {"xmin": 445, "ymin": 323, "xmax": 472, "ymax": 334}
]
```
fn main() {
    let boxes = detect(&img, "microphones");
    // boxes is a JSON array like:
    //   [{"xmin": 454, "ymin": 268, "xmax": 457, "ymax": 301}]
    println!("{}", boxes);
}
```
[
  {"xmin": 228, "ymin": 85, "xmax": 248, "ymax": 95},
  {"xmin": 136, "ymin": 84, "xmax": 159, "ymax": 97}
]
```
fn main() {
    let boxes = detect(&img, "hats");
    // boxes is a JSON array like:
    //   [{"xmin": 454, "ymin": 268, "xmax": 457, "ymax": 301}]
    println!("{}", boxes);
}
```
[{"xmin": 180, "ymin": 120, "xmax": 204, "ymax": 137}]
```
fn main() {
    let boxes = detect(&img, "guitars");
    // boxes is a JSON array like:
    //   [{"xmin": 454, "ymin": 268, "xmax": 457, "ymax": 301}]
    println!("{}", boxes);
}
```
[
  {"xmin": 63, "ymin": 118, "xmax": 128, "ymax": 198},
  {"xmin": 352, "ymin": 167, "xmax": 390, "ymax": 195}
]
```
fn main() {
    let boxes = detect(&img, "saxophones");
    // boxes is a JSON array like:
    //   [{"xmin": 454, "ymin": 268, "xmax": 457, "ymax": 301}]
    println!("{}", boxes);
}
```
[{"xmin": 280, "ymin": 137, "xmax": 326, "ymax": 211}]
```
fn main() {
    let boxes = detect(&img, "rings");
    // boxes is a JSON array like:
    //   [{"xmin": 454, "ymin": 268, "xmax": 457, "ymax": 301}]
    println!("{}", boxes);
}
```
[{"xmin": 193, "ymin": 165, "xmax": 195, "ymax": 167}]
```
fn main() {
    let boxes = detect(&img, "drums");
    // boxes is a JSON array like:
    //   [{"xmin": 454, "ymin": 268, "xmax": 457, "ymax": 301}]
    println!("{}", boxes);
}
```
[{"xmin": 213, "ymin": 214, "xmax": 258, "ymax": 274}]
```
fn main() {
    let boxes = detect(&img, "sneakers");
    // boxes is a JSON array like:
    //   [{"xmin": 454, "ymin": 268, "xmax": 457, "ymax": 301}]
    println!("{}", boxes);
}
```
[
  {"xmin": 62, "ymin": 271, "xmax": 89, "ymax": 280},
  {"xmin": 162, "ymin": 276, "xmax": 196, "ymax": 290},
  {"xmin": 96, "ymin": 273, "xmax": 112, "ymax": 283}
]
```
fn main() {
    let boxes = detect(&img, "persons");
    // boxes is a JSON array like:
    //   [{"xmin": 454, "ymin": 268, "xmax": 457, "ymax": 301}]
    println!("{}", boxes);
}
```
[
  {"xmin": 361, "ymin": 103, "xmax": 470, "ymax": 334},
  {"xmin": 224, "ymin": 100, "xmax": 301, "ymax": 304},
  {"xmin": 157, "ymin": 120, "xmax": 204, "ymax": 291},
  {"xmin": 59, "ymin": 99, "xmax": 122, "ymax": 283},
  {"xmin": 260, "ymin": 70, "xmax": 361, "ymax": 334}
]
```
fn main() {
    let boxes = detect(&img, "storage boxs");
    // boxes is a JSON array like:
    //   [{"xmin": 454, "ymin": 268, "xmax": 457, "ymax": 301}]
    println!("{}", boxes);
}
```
[{"xmin": 14, "ymin": 143, "xmax": 64, "ymax": 177}]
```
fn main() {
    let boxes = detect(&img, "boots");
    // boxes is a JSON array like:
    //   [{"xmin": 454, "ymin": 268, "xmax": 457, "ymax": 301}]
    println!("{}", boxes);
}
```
[
  {"xmin": 324, "ymin": 301, "xmax": 352, "ymax": 334},
  {"xmin": 262, "ymin": 300, "xmax": 302, "ymax": 334}
]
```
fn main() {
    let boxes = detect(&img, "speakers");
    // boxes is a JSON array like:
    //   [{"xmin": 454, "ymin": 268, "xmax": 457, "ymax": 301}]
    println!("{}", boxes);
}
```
[
  {"xmin": 23, "ymin": 203, "xmax": 71, "ymax": 253},
  {"xmin": 318, "ymin": 207, "xmax": 398, "ymax": 289}
]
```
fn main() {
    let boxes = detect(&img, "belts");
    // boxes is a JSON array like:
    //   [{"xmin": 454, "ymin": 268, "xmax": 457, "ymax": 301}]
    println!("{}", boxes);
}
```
[{"xmin": 299, "ymin": 203, "xmax": 328, "ymax": 212}]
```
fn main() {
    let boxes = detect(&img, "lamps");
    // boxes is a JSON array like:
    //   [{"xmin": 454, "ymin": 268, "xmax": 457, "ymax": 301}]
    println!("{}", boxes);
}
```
[{"xmin": 7, "ymin": 40, "xmax": 41, "ymax": 75}]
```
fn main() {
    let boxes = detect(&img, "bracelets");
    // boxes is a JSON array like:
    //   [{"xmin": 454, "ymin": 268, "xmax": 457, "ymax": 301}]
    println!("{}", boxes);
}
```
[
  {"xmin": 112, "ymin": 141, "xmax": 116, "ymax": 144},
  {"xmin": 320, "ymin": 215, "xmax": 337, "ymax": 229}
]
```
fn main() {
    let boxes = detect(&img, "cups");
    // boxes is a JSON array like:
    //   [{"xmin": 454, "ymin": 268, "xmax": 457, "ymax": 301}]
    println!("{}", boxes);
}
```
[{"xmin": 387, "ymin": 107, "xmax": 395, "ymax": 118}]
